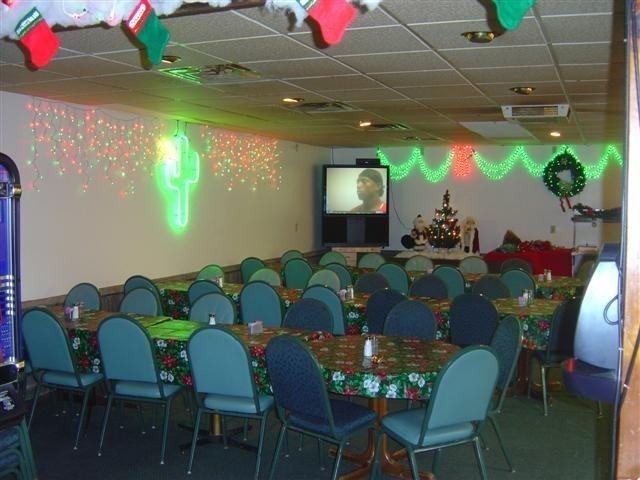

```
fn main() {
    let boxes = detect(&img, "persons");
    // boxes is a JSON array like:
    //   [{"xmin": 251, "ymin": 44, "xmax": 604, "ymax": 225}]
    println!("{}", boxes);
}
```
[
  {"xmin": 410, "ymin": 214, "xmax": 429, "ymax": 252},
  {"xmin": 459, "ymin": 217, "xmax": 480, "ymax": 255},
  {"xmin": 349, "ymin": 169, "xmax": 386, "ymax": 214}
]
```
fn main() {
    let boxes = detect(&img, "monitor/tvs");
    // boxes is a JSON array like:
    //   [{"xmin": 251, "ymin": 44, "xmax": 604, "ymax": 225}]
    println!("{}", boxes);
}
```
[{"xmin": 322, "ymin": 164, "xmax": 388, "ymax": 216}]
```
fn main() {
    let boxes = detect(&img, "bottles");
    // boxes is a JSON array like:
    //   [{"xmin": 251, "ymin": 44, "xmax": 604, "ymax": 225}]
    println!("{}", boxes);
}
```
[
  {"xmin": 362, "ymin": 336, "xmax": 379, "ymax": 358},
  {"xmin": 518, "ymin": 269, "xmax": 552, "ymax": 306},
  {"xmin": 208, "ymin": 312, "xmax": 215, "ymax": 326},
  {"xmin": 338, "ymin": 285, "xmax": 356, "ymax": 301},
  {"xmin": 65, "ymin": 302, "xmax": 85, "ymax": 321},
  {"xmin": 211, "ymin": 275, "xmax": 225, "ymax": 296}
]
